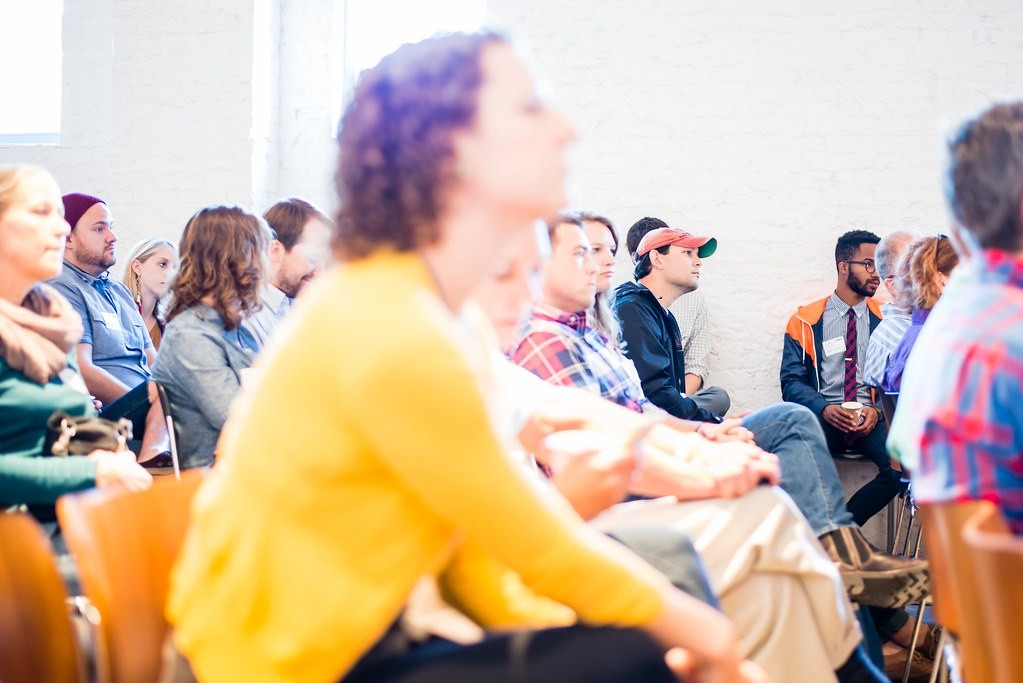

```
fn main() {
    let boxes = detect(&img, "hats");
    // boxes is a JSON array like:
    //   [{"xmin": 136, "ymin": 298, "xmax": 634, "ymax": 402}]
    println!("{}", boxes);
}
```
[
  {"xmin": 63, "ymin": 193, "xmax": 106, "ymax": 230},
  {"xmin": 635, "ymin": 228, "xmax": 717, "ymax": 262}
]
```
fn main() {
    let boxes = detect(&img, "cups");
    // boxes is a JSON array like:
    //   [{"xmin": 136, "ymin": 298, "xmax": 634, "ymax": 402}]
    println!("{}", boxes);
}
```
[{"xmin": 841, "ymin": 401, "xmax": 864, "ymax": 427}]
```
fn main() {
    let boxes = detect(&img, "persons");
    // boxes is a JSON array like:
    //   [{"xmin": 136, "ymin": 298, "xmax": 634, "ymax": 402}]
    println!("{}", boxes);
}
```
[
  {"xmin": 0, "ymin": 159, "xmax": 154, "ymax": 656},
  {"xmin": 41, "ymin": 194, "xmax": 338, "ymax": 469},
  {"xmin": 883, "ymin": 101, "xmax": 1023, "ymax": 538},
  {"xmin": 780, "ymin": 231, "xmax": 959, "ymax": 526},
  {"xmin": 158, "ymin": 27, "xmax": 765, "ymax": 683},
  {"xmin": 404, "ymin": 213, "xmax": 932, "ymax": 683}
]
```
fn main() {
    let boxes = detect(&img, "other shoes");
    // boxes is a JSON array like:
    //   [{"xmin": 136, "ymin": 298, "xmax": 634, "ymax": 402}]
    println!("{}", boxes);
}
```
[
  {"xmin": 886, "ymin": 650, "xmax": 934, "ymax": 682},
  {"xmin": 914, "ymin": 621, "xmax": 942, "ymax": 658}
]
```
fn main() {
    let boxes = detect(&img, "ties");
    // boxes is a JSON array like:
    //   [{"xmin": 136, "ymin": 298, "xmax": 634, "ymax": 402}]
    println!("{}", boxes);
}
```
[{"xmin": 844, "ymin": 308, "xmax": 857, "ymax": 402}]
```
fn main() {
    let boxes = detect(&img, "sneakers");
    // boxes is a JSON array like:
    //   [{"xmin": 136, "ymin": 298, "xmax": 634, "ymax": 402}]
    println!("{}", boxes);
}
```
[{"xmin": 816, "ymin": 527, "xmax": 931, "ymax": 609}]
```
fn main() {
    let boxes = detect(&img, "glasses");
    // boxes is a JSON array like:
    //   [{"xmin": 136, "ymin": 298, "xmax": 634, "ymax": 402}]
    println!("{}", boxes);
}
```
[{"xmin": 846, "ymin": 261, "xmax": 876, "ymax": 273}]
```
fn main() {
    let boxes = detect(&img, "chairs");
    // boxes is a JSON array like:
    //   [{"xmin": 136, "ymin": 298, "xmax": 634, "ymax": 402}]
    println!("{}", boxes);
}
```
[
  {"xmin": 0, "ymin": 510, "xmax": 88, "ymax": 683},
  {"xmin": 888, "ymin": 442, "xmax": 1023, "ymax": 683},
  {"xmin": 60, "ymin": 466, "xmax": 208, "ymax": 683}
]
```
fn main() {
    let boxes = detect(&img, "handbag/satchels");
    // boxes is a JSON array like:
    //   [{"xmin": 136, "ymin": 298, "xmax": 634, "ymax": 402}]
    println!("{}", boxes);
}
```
[{"xmin": 31, "ymin": 414, "xmax": 133, "ymax": 524}]
